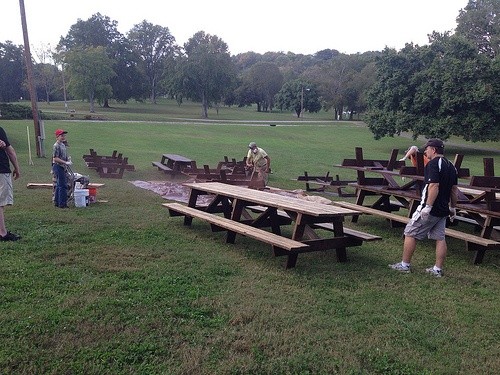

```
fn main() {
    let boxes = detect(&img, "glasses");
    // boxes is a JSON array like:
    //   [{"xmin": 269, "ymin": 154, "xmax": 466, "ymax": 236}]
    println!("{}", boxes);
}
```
[{"xmin": 249, "ymin": 147, "xmax": 253, "ymax": 149}]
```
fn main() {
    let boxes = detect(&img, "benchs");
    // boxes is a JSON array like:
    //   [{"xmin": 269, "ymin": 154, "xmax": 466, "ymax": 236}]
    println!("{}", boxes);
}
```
[
  {"xmin": 152, "ymin": 161, "xmax": 173, "ymax": 173},
  {"xmin": 333, "ymin": 164, "xmax": 500, "ymax": 193},
  {"xmin": 246, "ymin": 205, "xmax": 384, "ymax": 242},
  {"xmin": 291, "ymin": 179, "xmax": 345, "ymax": 188},
  {"xmin": 334, "ymin": 200, "xmax": 500, "ymax": 249},
  {"xmin": 160, "ymin": 202, "xmax": 311, "ymax": 253}
]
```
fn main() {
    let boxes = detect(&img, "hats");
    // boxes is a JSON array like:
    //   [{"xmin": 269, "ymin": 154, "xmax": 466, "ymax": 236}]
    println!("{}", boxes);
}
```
[
  {"xmin": 55, "ymin": 128, "xmax": 68, "ymax": 137},
  {"xmin": 422, "ymin": 138, "xmax": 444, "ymax": 149},
  {"xmin": 249, "ymin": 142, "xmax": 257, "ymax": 147}
]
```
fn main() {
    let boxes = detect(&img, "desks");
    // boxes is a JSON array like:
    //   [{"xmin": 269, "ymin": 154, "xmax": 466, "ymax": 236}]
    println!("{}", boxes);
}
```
[
  {"xmin": 299, "ymin": 147, "xmax": 500, "ymax": 264},
  {"xmin": 169, "ymin": 180, "xmax": 362, "ymax": 268},
  {"xmin": 81, "ymin": 148, "xmax": 135, "ymax": 178},
  {"xmin": 161, "ymin": 153, "xmax": 272, "ymax": 185}
]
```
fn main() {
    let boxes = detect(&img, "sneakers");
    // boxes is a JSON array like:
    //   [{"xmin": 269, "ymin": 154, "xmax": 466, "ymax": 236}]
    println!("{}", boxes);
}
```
[
  {"xmin": 388, "ymin": 262, "xmax": 411, "ymax": 274},
  {"xmin": 425, "ymin": 267, "xmax": 442, "ymax": 277}
]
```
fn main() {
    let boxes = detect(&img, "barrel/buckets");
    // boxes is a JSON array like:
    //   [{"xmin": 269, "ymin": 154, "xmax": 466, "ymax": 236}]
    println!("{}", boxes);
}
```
[
  {"xmin": 84, "ymin": 188, "xmax": 96, "ymax": 203},
  {"xmin": 74, "ymin": 189, "xmax": 90, "ymax": 208}
]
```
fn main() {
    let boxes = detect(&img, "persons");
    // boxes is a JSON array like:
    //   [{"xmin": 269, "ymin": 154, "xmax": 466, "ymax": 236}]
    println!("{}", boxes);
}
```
[
  {"xmin": 51, "ymin": 129, "xmax": 72, "ymax": 208},
  {"xmin": 399, "ymin": 146, "xmax": 430, "ymax": 167},
  {"xmin": 246, "ymin": 142, "xmax": 270, "ymax": 191},
  {"xmin": 388, "ymin": 138, "xmax": 458, "ymax": 277},
  {"xmin": 0, "ymin": 114, "xmax": 23, "ymax": 241}
]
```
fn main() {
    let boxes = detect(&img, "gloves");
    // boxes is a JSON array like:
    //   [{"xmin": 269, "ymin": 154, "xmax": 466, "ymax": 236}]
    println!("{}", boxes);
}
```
[
  {"xmin": 448, "ymin": 206, "xmax": 456, "ymax": 222},
  {"xmin": 420, "ymin": 205, "xmax": 433, "ymax": 220},
  {"xmin": 66, "ymin": 161, "xmax": 72, "ymax": 165}
]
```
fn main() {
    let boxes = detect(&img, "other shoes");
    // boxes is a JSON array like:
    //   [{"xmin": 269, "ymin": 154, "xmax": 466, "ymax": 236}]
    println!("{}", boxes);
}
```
[
  {"xmin": 61, "ymin": 206, "xmax": 69, "ymax": 208},
  {"xmin": 55, "ymin": 204, "xmax": 57, "ymax": 207},
  {"xmin": 0, "ymin": 231, "xmax": 22, "ymax": 242}
]
